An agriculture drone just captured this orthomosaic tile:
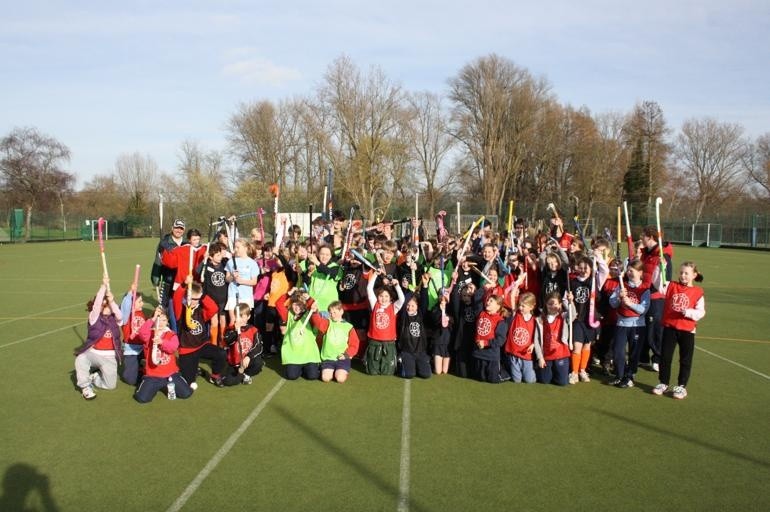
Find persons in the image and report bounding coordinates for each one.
[73,272,124,401]
[133,305,194,404]
[650,256,707,401]
[151,207,673,391]
[119,285,149,387]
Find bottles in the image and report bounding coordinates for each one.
[167,376,178,401]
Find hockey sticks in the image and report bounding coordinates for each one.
[151,282,166,366]
[565,266,575,350]
[570,196,579,237]
[445,198,514,292]
[309,203,312,272]
[200,215,212,284]
[218,215,240,287]
[280,216,286,241]
[588,257,600,329]
[98,217,110,293]
[604,227,627,310]
[340,191,422,286]
[517,218,529,291]
[208,210,267,227]
[235,293,244,374]
[573,214,590,252]
[185,245,197,330]
[129,265,140,339]
[655,197,666,289]
[546,202,563,234]
[440,253,449,328]
[300,274,330,336]
[257,207,266,268]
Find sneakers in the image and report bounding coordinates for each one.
[650,383,669,396]
[209,376,225,388]
[672,385,688,400]
[568,372,579,385]
[81,384,97,401]
[650,363,659,372]
[242,374,253,385]
[89,369,101,383]
[594,367,610,380]
[614,376,635,389]
[579,371,591,383]
[607,375,626,386]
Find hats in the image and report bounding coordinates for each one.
[172,217,185,229]
